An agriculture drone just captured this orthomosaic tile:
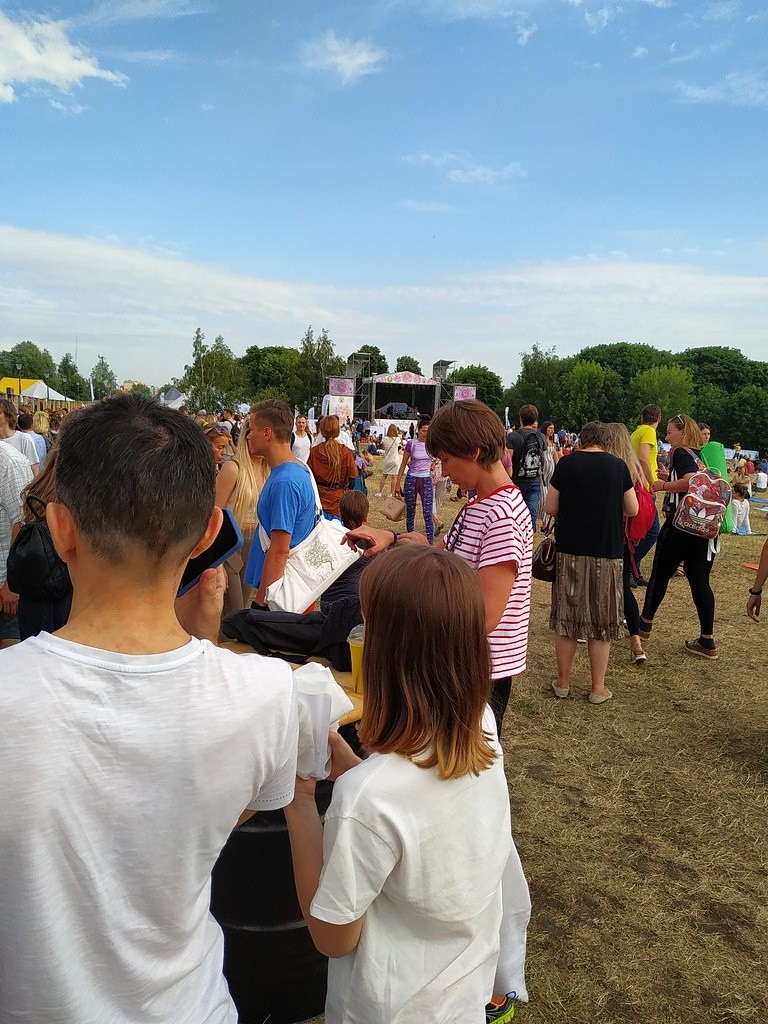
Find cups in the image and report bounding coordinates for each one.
[346,624,364,694]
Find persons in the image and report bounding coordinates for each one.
[284,545,510,1024]
[1,394,301,1024]
[545,421,640,705]
[1,398,768,664]
[341,399,532,1024]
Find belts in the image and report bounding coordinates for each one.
[316,481,341,489]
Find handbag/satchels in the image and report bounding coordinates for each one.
[263,515,366,615]
[379,491,406,522]
[532,532,556,582]
[220,608,326,664]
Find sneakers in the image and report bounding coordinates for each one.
[485,995,515,1024]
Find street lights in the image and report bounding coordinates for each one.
[44,369,51,406]
[86,383,108,403]
[61,374,67,405]
[15,358,24,405]
[76,380,80,407]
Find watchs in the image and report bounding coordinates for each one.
[749,587,762,595]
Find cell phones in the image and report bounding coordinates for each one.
[347,532,376,550]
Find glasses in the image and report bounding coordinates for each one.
[676,412,685,425]
[208,426,229,433]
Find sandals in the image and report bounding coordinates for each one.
[629,643,647,665]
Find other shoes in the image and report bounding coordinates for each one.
[588,685,612,704]
[685,638,718,659]
[434,521,444,537]
[374,490,381,497]
[552,678,570,697]
[576,635,587,643]
[623,619,650,641]
[446,490,450,496]
[630,572,649,588]
[390,493,392,496]
[674,563,686,577]
[450,497,459,502]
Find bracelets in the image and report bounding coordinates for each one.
[663,481,666,491]
[389,530,398,547]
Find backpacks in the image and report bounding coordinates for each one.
[668,445,733,539]
[7,496,71,597]
[622,463,658,579]
[515,429,544,480]
[226,419,241,447]
